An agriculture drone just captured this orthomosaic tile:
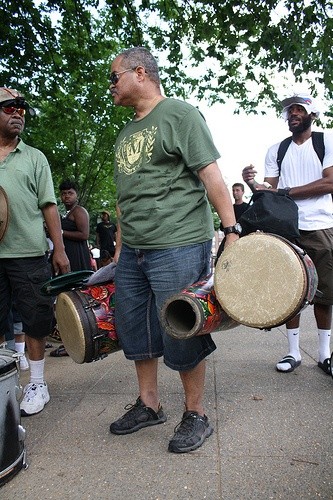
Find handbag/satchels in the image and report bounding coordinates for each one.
[236,189,301,239]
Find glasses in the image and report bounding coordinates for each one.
[109,68,147,85]
[2,106,26,116]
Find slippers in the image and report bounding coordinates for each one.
[276,356,301,373]
[317,357,332,375]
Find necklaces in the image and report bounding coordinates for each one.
[62,203,77,219]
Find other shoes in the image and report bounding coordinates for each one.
[50,346,69,356]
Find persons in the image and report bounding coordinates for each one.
[107,47,240,453]
[242,94,333,376]
[0,87,71,416]
[12,179,117,371]
[220,183,250,231]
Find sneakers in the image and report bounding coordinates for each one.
[168,411,213,452]
[109,396,167,434]
[19,382,51,416]
[20,358,29,370]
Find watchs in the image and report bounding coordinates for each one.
[224,223,242,235]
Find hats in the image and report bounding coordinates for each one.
[0,87,29,107]
[281,91,320,114]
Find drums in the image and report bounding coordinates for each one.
[0,342,29,488]
[54,280,123,364]
[0,185,10,242]
[160,273,240,340]
[215,231,318,330]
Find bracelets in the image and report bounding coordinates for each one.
[284,187,290,195]
